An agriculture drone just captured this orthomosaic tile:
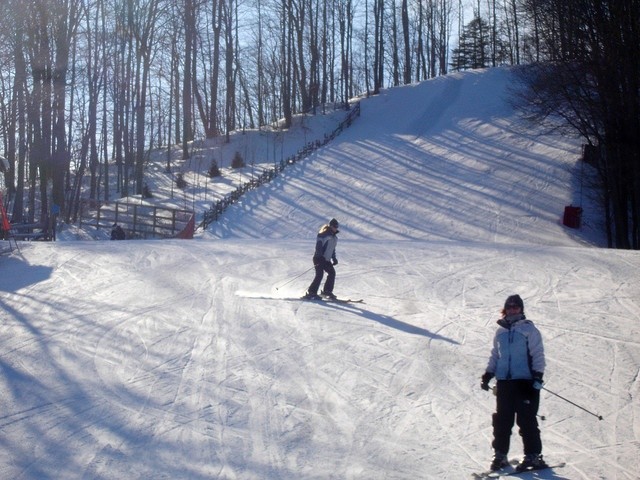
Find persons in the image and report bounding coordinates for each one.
[110,223,126,240]
[305,218,340,302]
[481,294,546,472]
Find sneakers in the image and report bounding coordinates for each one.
[323,291,337,299]
[309,293,322,300]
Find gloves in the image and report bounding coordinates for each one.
[481,373,492,391]
[534,373,543,390]
[331,258,338,267]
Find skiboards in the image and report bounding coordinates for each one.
[283,297,364,303]
[471,459,566,478]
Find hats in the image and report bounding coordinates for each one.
[506,294,522,305]
[329,218,338,226]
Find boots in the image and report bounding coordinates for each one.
[490,449,509,470]
[518,451,545,470]
